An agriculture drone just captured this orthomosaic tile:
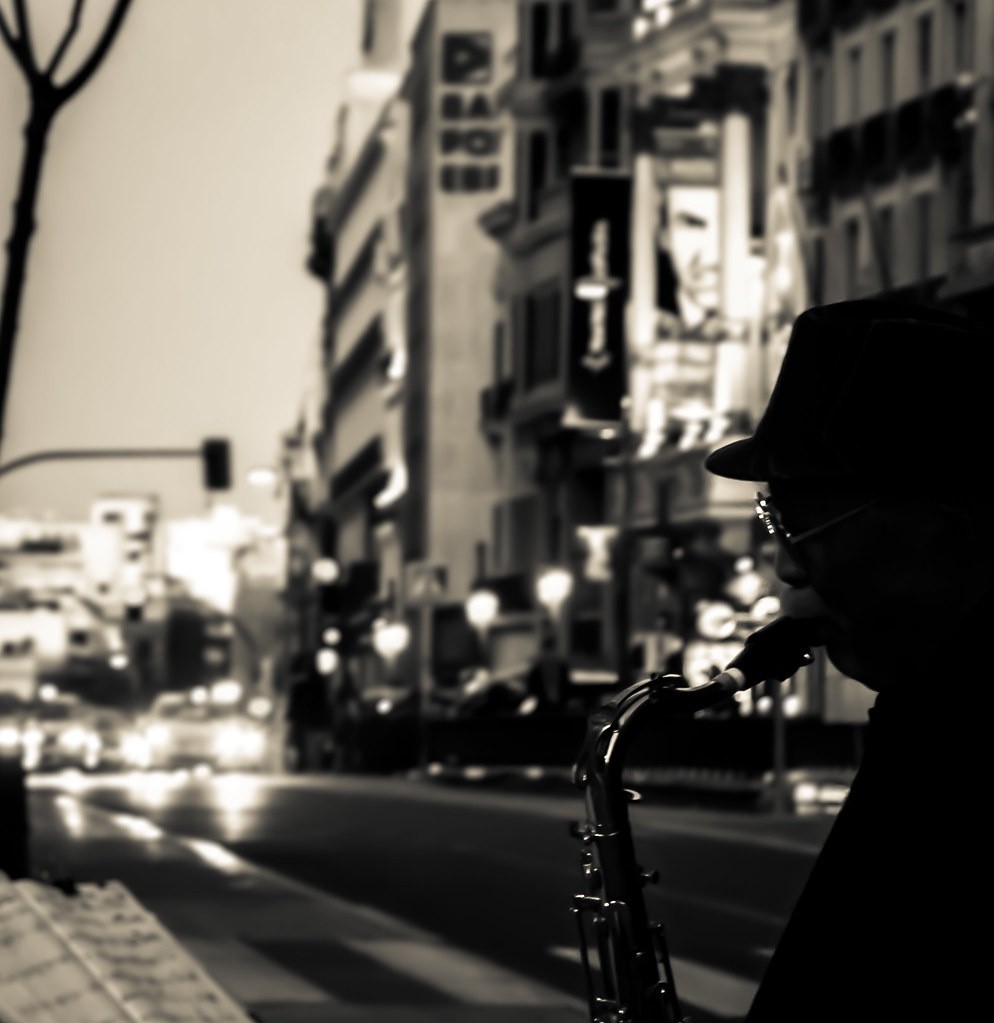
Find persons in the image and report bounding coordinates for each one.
[706,281,994,1023]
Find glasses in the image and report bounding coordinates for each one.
[754,488,866,545]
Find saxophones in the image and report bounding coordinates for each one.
[563,609,828,1023]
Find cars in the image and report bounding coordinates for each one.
[0,689,265,774]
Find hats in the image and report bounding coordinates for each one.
[705,297,994,481]
